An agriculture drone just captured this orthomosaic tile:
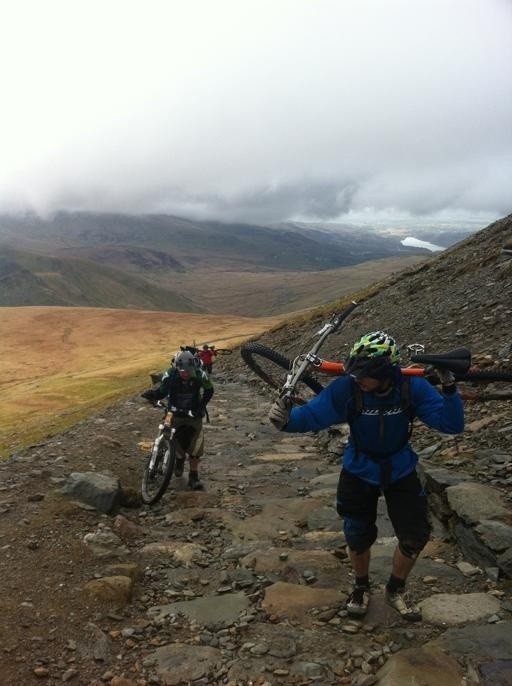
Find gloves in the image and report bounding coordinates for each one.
[433,349,455,387]
[269,397,294,431]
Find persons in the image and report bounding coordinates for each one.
[266,330,465,621]
[141,350,215,491]
[196,344,217,375]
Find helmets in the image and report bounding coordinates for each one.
[343,330,402,379]
[175,350,199,372]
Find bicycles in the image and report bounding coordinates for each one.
[136,392,202,509]
[181,340,232,358]
[234,294,511,439]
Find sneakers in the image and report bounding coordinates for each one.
[382,584,422,621]
[189,479,203,490]
[346,587,371,615]
[175,452,185,477]
